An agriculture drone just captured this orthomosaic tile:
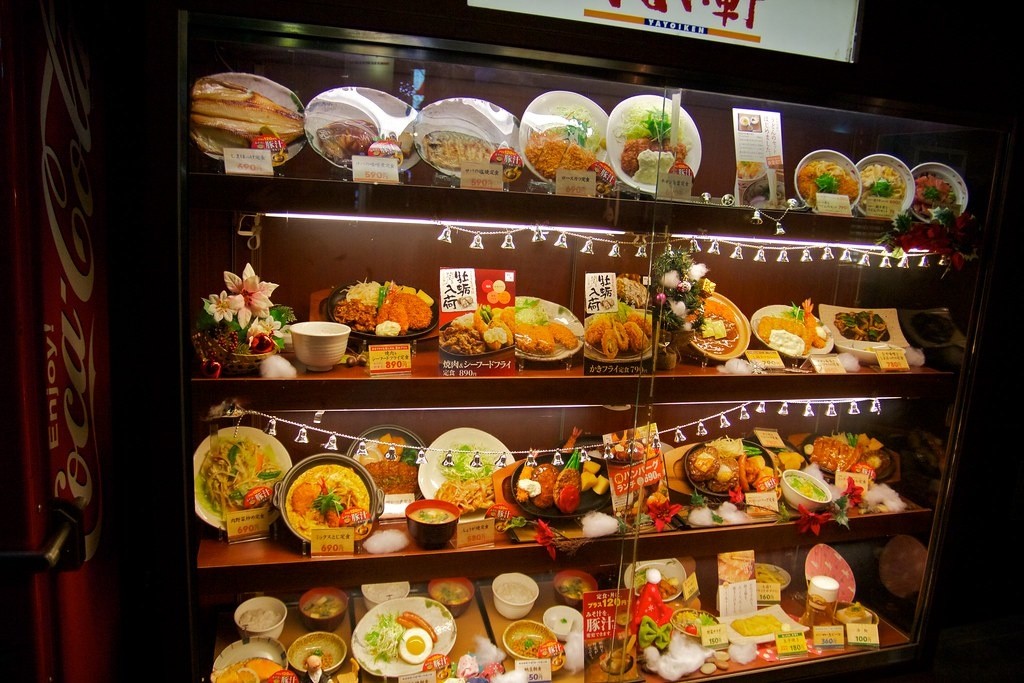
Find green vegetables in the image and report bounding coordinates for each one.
[441,444,494,480]
[791,479,828,502]
[696,613,715,634]
[312,488,345,518]
[228,441,281,499]
[364,611,405,662]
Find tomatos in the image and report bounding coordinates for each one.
[686,624,697,635]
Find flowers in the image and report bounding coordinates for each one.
[201,262,297,355]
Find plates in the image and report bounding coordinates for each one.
[191,73,701,193]
[319,281,440,340]
[624,543,879,648]
[439,319,516,362]
[212,638,288,683]
[674,432,896,497]
[351,596,457,677]
[583,308,652,363]
[686,292,964,365]
[193,425,610,541]
[605,438,662,464]
[514,296,586,361]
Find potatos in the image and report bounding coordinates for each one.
[580,461,610,495]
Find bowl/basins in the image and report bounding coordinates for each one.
[405,498,461,550]
[493,568,598,661]
[234,596,287,639]
[781,469,832,512]
[737,164,785,210]
[288,632,347,677]
[299,588,349,631]
[428,575,475,619]
[793,149,968,223]
[290,321,352,372]
[361,582,410,610]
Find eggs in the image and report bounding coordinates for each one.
[398,627,433,664]
[646,568,661,584]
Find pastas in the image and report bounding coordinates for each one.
[201,438,279,514]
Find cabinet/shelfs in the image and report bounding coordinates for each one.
[91,0,1004,683]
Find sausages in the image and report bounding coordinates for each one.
[398,611,438,642]
